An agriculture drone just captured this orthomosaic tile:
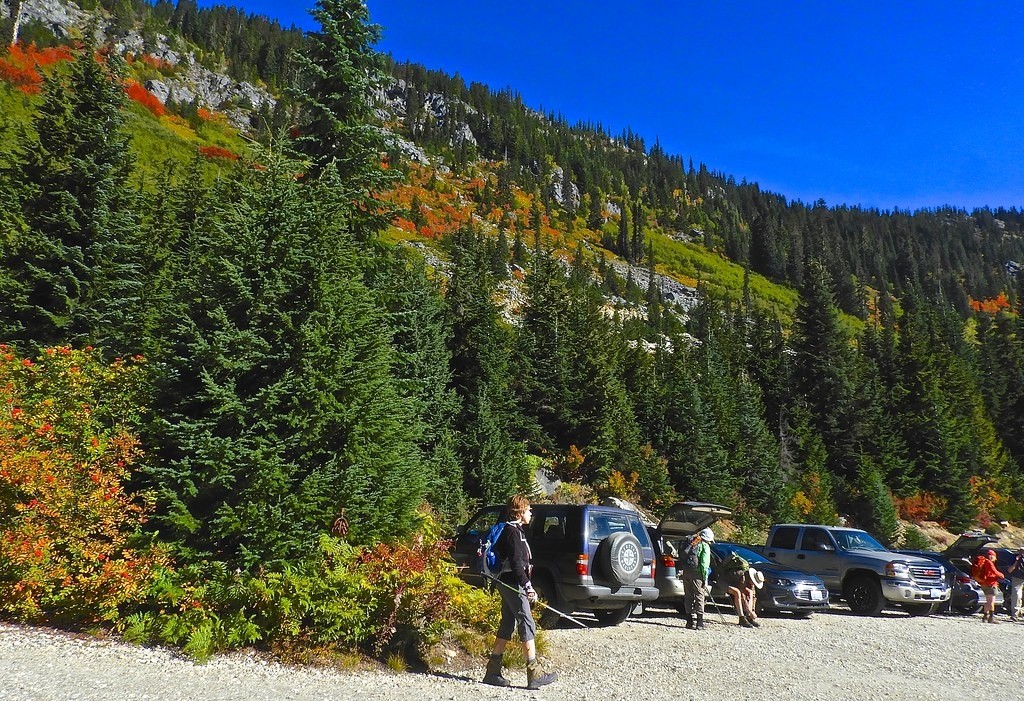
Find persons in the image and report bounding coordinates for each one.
[979,550,1006,623]
[482,495,558,690]
[710,555,765,629]
[678,527,716,630]
[1007,546,1024,622]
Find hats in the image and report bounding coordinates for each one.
[749,567,764,589]
[699,526,715,544]
[986,550,996,557]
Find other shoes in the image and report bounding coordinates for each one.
[1009,616,1019,621]
[982,614,987,622]
[988,616,999,624]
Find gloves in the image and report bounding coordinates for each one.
[522,581,538,603]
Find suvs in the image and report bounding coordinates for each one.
[444,501,660,630]
[763,523,952,617]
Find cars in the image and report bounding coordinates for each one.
[614,501,734,614]
[707,542,829,618]
[974,546,1024,614]
[887,527,1005,616]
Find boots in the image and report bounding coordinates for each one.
[525,658,558,689]
[685,614,693,629]
[746,616,760,627]
[696,614,704,630]
[739,616,753,628]
[483,654,510,687]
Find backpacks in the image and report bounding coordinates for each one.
[470,521,522,575]
[972,555,991,585]
[678,536,701,569]
[716,551,750,576]
